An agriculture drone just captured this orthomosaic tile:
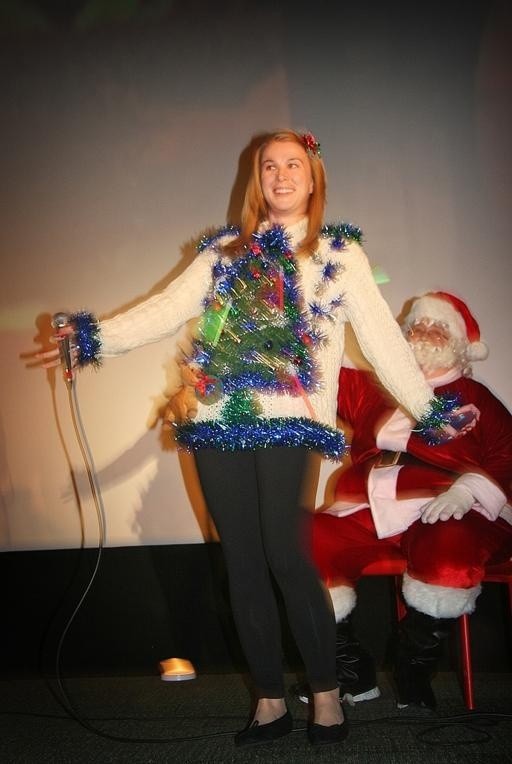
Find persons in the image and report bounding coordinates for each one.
[27,126,482,752]
[289,289,509,712]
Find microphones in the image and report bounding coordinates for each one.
[50,312,72,383]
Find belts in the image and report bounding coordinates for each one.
[360,448,429,470]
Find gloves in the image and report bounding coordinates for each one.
[419,486,471,525]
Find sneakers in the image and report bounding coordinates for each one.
[392,657,438,713]
[292,667,382,705]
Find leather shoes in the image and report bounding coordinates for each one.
[306,699,349,748]
[233,702,294,746]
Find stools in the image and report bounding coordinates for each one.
[358,547,512,714]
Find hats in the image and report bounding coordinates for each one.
[405,290,489,364]
